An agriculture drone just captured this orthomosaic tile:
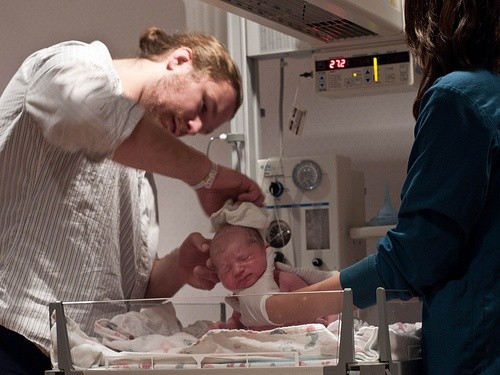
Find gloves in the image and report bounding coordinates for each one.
[275,262,338,286]
[224,247,285,327]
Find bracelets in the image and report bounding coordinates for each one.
[189,161,219,191]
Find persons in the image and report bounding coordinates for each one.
[224,0,500,375]
[207,224,330,332]
[0,25,264,375]
